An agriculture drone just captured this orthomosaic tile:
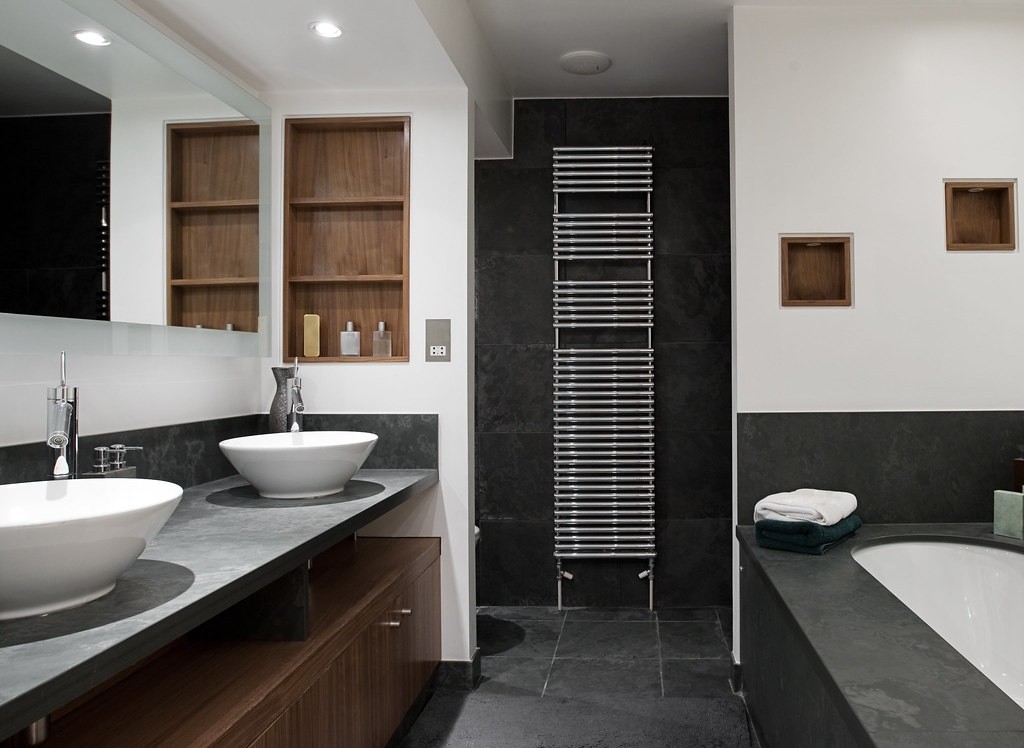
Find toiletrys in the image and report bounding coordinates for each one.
[339,321,362,357]
[108,444,144,479]
[371,321,392,358]
[82,446,122,478]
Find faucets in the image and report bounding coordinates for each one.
[286,357,306,433]
[43,349,81,480]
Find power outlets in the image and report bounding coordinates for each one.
[426,319,452,362]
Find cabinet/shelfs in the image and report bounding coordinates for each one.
[283,117,409,363]
[162,117,261,333]
[35,538,443,748]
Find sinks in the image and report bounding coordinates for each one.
[218,429,379,500]
[1,476,184,624]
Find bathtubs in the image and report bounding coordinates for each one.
[733,505,1024,748]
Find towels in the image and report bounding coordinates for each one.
[752,516,864,555]
[752,487,858,526]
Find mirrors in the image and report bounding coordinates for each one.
[1,0,261,333]
